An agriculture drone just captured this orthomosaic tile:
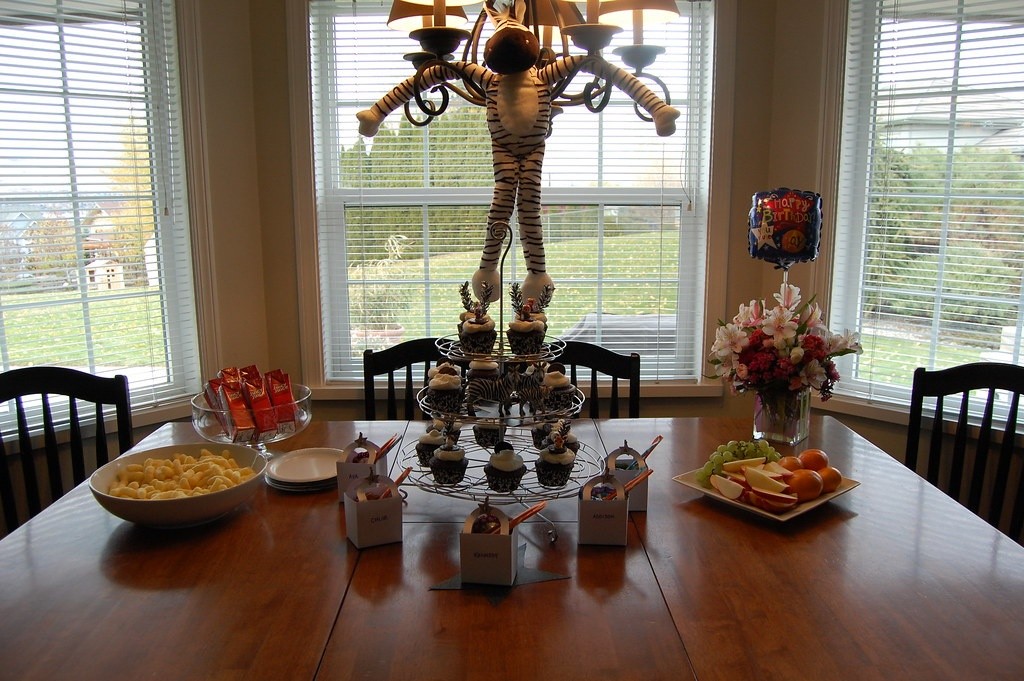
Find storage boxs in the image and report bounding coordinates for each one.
[335,430,650,588]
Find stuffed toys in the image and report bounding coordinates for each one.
[356,18,682,311]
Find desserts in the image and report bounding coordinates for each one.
[427,357,576,414]
[415,416,580,493]
[456,280,553,355]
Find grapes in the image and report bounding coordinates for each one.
[695,435,781,489]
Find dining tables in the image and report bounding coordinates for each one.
[0,421,1021,681]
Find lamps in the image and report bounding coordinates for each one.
[386,0,681,138]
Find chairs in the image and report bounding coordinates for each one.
[904,360,1024,546]
[544,340,640,417]
[364,337,473,423]
[0,364,134,536]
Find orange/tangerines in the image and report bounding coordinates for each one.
[779,448,842,502]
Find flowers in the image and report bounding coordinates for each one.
[702,281,863,432]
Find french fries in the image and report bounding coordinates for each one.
[107,448,256,500]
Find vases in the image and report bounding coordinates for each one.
[752,384,811,445]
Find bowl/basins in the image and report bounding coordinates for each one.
[191,382,313,458]
[87,442,267,530]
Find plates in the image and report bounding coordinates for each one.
[264,447,344,492]
[670,456,861,524]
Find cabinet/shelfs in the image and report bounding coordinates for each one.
[395,220,608,545]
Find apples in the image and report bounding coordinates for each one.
[710,456,798,513]
